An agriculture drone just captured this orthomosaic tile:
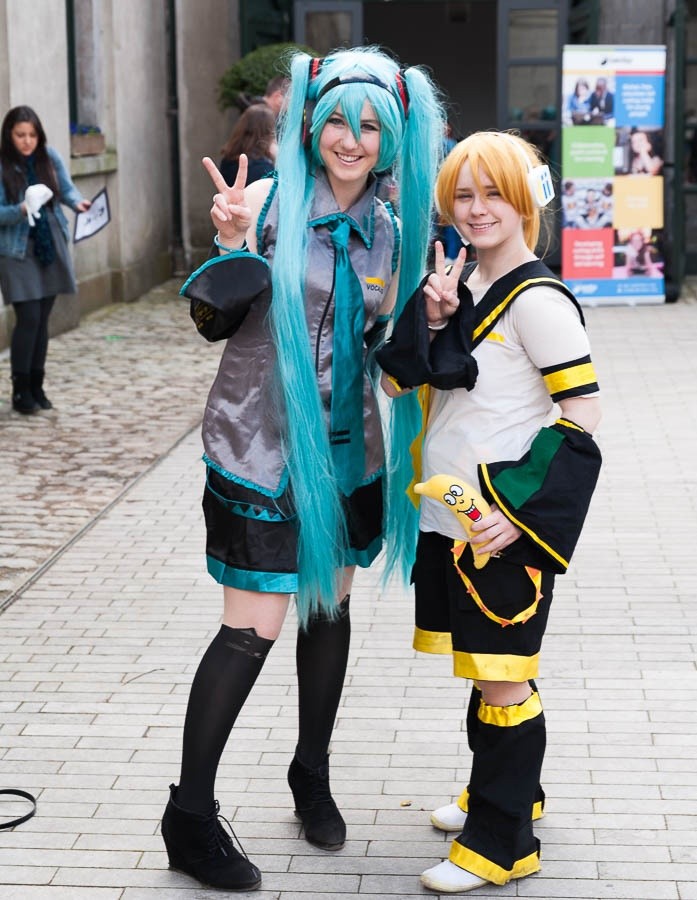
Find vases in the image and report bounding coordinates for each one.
[71,135,106,158]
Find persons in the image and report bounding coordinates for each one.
[629,133,664,175]
[562,180,612,227]
[0,106,93,413]
[220,102,274,189]
[159,49,443,888]
[388,132,600,896]
[252,75,288,116]
[570,79,613,126]
[623,232,663,277]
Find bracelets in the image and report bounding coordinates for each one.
[428,320,449,331]
[214,235,247,254]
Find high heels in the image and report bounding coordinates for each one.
[288,743,346,850]
[162,781,261,891]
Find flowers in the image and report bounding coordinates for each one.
[70,124,101,135]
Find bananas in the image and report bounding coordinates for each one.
[413,473,492,569]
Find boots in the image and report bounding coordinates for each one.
[11,375,40,416]
[34,371,51,409]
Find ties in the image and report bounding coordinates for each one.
[328,217,368,498]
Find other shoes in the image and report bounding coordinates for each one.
[430,801,470,831]
[421,860,489,892]
